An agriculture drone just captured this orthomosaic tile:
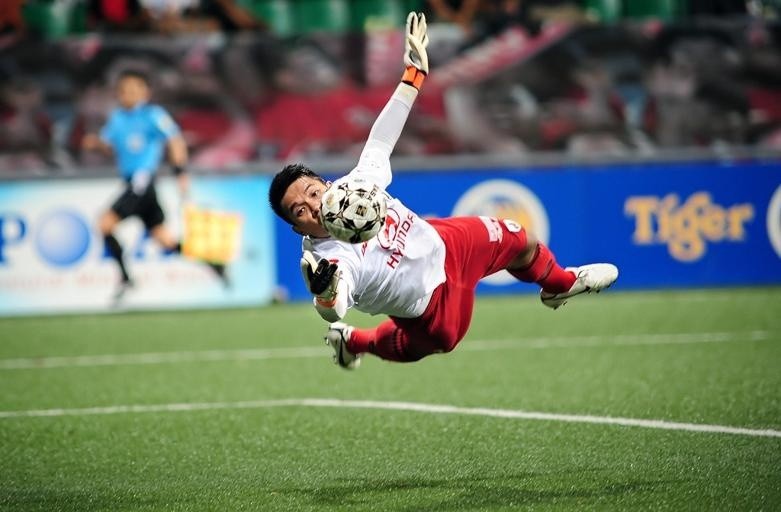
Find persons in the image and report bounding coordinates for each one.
[83,72,234,306]
[268,11,620,369]
[1,0,780,177]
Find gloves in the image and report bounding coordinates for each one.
[403,12,429,76]
[300,250,342,301]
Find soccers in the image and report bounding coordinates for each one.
[320,178,388,243]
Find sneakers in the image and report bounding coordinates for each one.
[540,263,619,309]
[324,322,361,370]
